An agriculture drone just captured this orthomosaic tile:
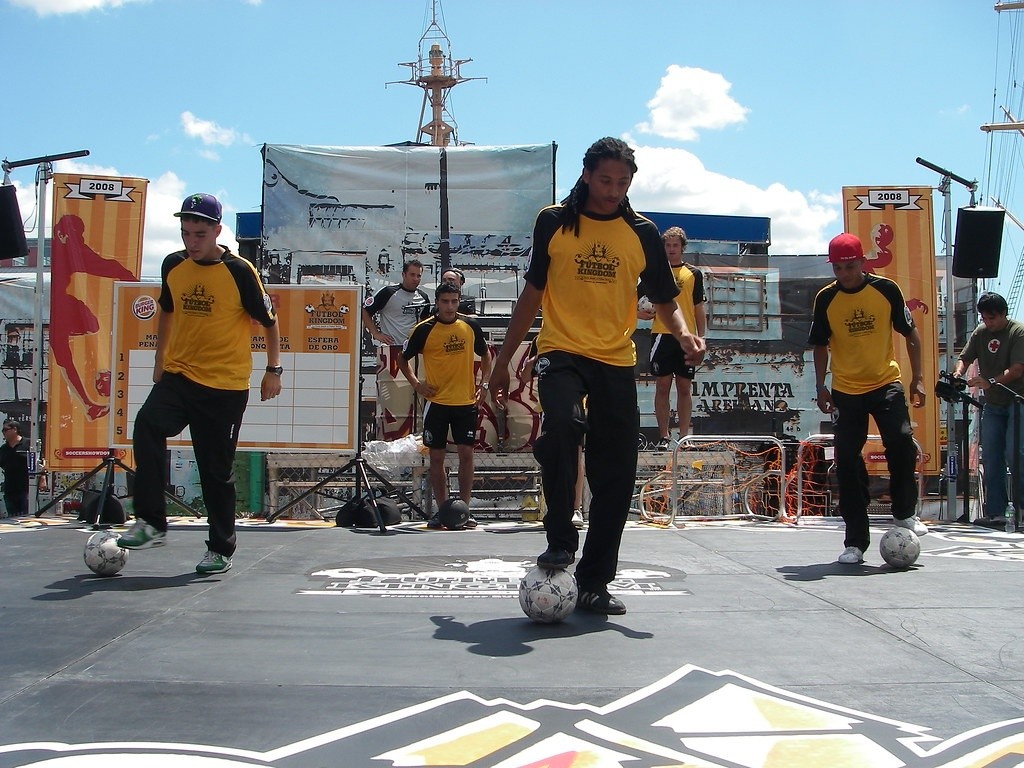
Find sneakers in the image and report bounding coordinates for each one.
[577,581,627,614]
[537,547,576,569]
[895,514,928,537]
[839,547,863,562]
[196,550,233,573]
[117,518,167,550]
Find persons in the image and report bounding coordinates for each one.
[636,227,706,451]
[430,269,474,316]
[363,260,430,345]
[116,193,283,574]
[490,136,706,615]
[953,291,1024,531]
[396,283,492,528]
[0,421,31,517]
[520,334,587,526]
[807,233,928,564]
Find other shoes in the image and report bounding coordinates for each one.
[427,512,444,527]
[465,513,477,527]
[571,506,583,525]
[974,515,1007,527]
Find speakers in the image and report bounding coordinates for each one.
[952,205,1005,278]
[0,185,30,260]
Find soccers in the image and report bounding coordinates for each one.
[879,525,922,568]
[439,498,470,529]
[519,564,579,625]
[84,531,129,576]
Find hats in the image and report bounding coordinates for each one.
[442,268,465,285]
[826,232,865,263]
[174,192,222,221]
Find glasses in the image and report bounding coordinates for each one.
[2,428,13,432]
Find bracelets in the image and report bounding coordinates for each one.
[816,385,827,392]
[701,336,705,339]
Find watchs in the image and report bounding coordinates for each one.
[987,377,996,388]
[479,382,489,389]
[266,366,283,376]
